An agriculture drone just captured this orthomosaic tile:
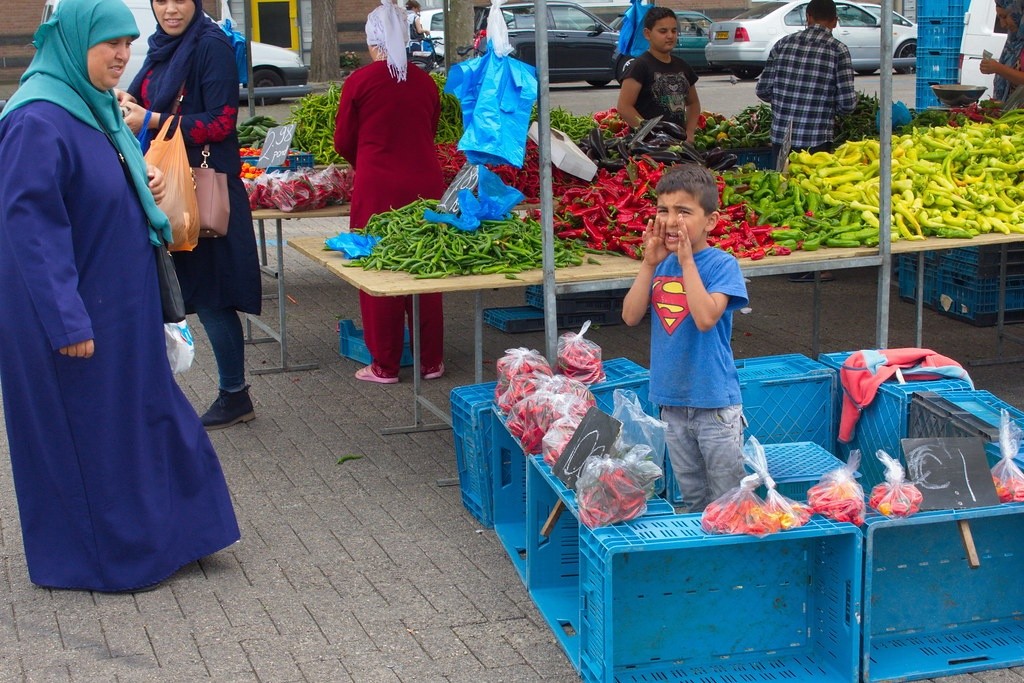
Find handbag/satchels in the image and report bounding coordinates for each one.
[990,407,1024,503]
[700,473,781,539]
[557,319,606,385]
[143,115,200,252]
[409,15,424,39]
[241,162,354,213]
[868,449,923,520]
[806,449,866,527]
[495,346,669,523]
[741,435,815,531]
[192,144,231,237]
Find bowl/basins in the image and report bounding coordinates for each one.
[929,81,988,105]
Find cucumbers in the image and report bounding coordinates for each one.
[236,114,283,149]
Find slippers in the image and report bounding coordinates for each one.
[355,365,399,383]
[423,361,445,380]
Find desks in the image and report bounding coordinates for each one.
[281,188,1024,490]
[252,189,350,277]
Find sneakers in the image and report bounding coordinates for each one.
[199,384,255,430]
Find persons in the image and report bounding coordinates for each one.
[617,7,701,144]
[113,0,263,431]
[0,0,243,592]
[980,0,1024,102]
[334,0,447,384]
[403,0,431,52]
[756,0,857,281]
[622,164,751,514]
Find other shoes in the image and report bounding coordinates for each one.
[787,271,834,283]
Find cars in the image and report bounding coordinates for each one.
[608,11,712,70]
[475,1,635,87]
[419,6,515,65]
[858,4,916,27]
[705,0,917,80]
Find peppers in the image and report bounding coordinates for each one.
[281,72,467,189]
[869,482,922,516]
[336,453,363,464]
[496,332,605,465]
[527,101,630,146]
[891,93,1024,242]
[237,146,356,213]
[578,443,664,529]
[324,154,620,281]
[695,87,881,154]
[520,133,881,260]
[701,484,864,536]
[991,474,1024,502]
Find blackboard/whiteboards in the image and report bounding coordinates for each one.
[256,124,296,168]
[901,438,1000,511]
[551,406,624,491]
[628,115,664,147]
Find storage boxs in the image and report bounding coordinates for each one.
[915,0,971,116]
[714,147,775,174]
[239,150,313,174]
[338,242,1024,683]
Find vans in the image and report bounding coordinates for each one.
[42,1,309,107]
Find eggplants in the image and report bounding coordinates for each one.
[578,115,737,174]
